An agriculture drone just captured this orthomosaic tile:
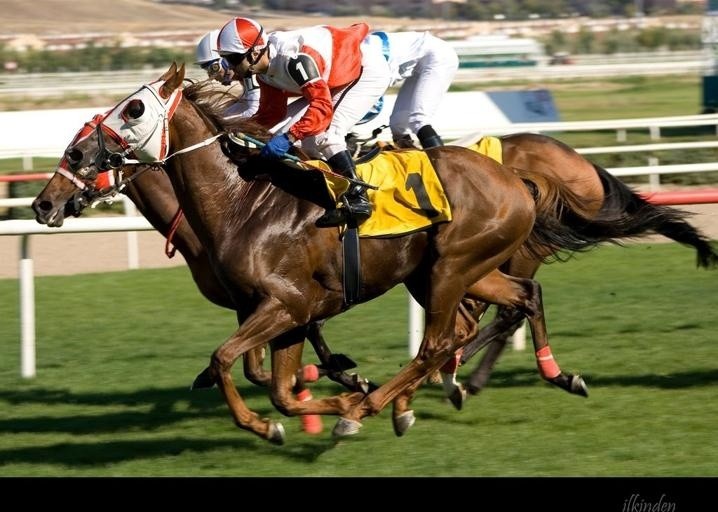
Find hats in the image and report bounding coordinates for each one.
[214,17,268,57]
[193,30,222,65]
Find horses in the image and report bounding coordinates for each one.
[28,105,718,399]
[63,58,700,446]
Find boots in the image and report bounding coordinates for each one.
[315,149,373,229]
[421,135,443,150]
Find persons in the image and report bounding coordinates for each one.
[212,16,392,229]
[191,26,460,149]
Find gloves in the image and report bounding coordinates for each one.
[262,132,292,160]
